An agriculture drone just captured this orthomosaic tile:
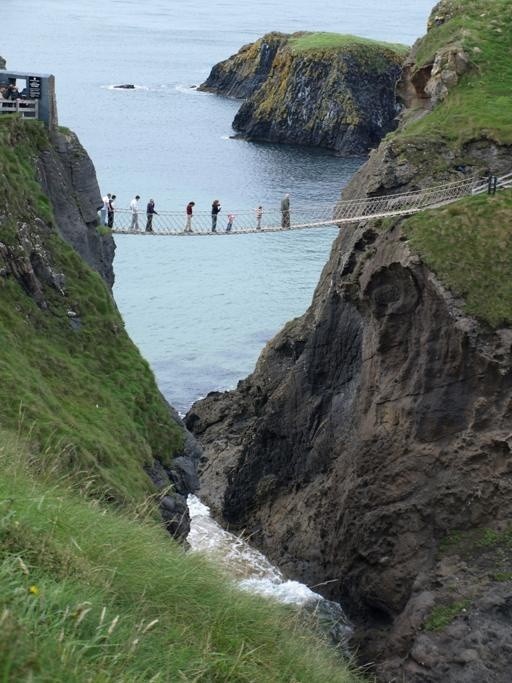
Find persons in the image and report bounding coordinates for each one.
[128,194,143,230]
[101,193,111,223]
[210,199,222,231]
[145,198,160,231]
[225,212,236,232]
[106,194,118,227]
[281,192,292,228]
[0,83,31,114]
[255,205,264,228]
[182,200,195,232]
